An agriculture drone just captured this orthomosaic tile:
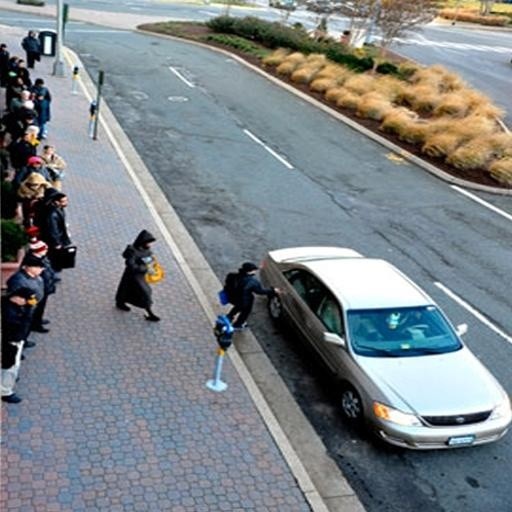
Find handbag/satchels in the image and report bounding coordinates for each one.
[144,255,163,282]
[48,245,78,269]
[219,290,228,304]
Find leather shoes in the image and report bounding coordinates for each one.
[1,275,61,405]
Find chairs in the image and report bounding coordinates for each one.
[323,303,359,336]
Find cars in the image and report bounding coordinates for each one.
[261,244,512,451]
[268,0,368,17]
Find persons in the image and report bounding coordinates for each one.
[113,228,160,322]
[218,262,275,330]
[0,43,77,402]
[21,30,41,68]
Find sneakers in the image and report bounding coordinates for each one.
[144,314,159,321]
[116,302,130,311]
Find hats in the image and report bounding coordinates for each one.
[29,236,48,254]
